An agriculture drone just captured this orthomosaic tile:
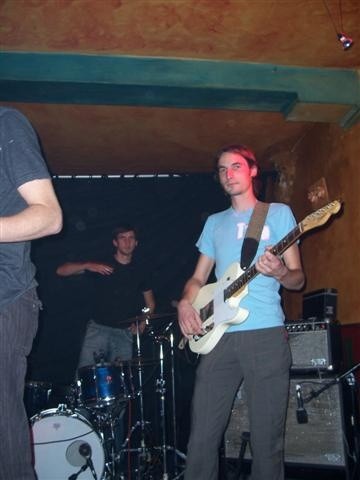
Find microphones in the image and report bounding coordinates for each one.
[79,444,90,457]
[238,431,250,471]
[295,383,308,425]
[161,319,175,334]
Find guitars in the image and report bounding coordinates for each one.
[188,200,341,355]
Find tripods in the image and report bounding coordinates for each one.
[102,317,188,480]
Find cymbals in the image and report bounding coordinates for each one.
[121,313,175,323]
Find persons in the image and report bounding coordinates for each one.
[55,226,156,382]
[176,144,305,480]
[0,104,63,480]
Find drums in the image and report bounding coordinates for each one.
[28,407,106,480]
[78,361,140,408]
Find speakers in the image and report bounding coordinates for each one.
[224,371,357,471]
